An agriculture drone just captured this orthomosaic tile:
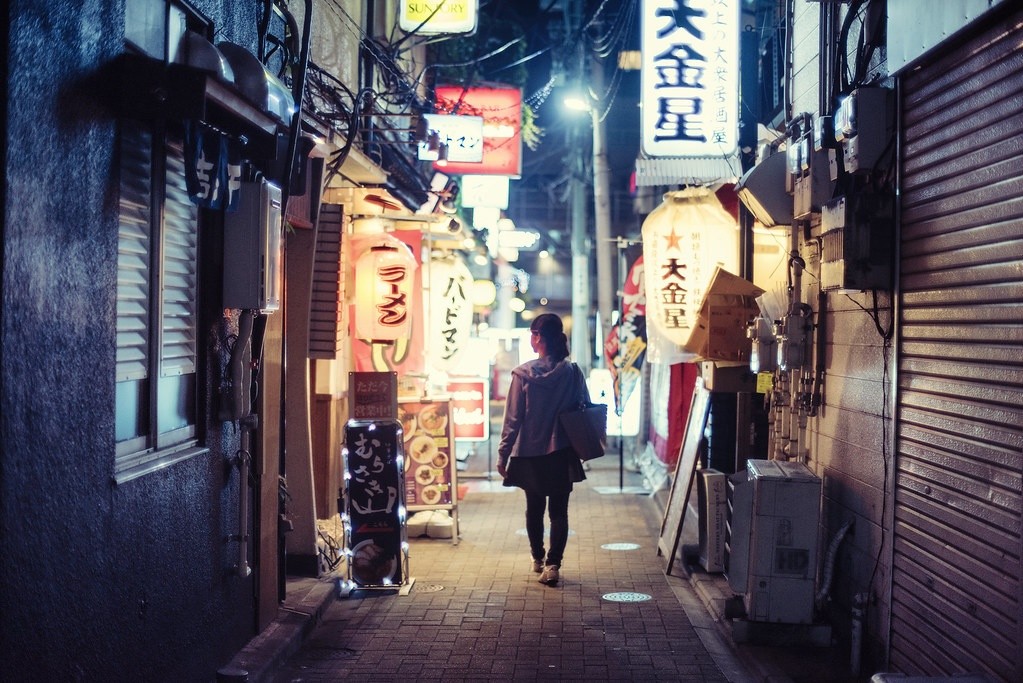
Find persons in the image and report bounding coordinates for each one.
[496,313,591,583]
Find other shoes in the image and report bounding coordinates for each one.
[531,559,545,572]
[535,565,560,584]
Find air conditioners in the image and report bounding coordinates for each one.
[732,452,827,626]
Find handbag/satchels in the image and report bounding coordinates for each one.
[559,362,608,461]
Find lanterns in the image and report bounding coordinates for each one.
[354,246,411,345]
[643,186,739,348]
[421,255,475,369]
